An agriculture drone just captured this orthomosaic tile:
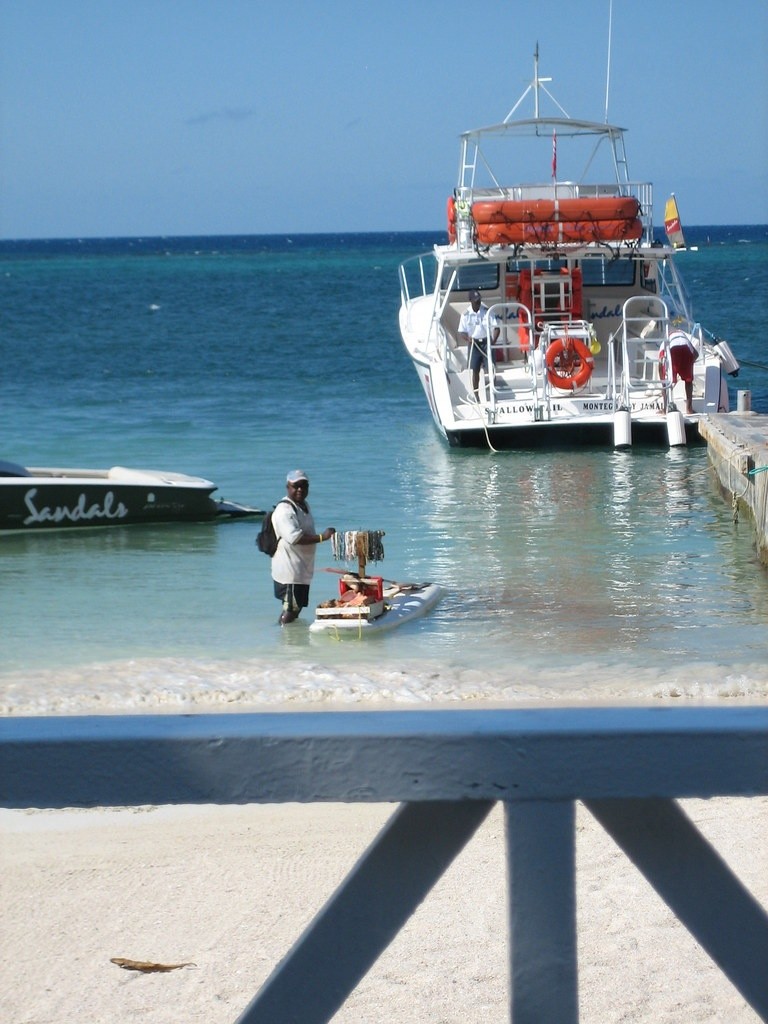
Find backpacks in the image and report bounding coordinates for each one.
[256,500,298,558]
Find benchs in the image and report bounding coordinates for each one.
[610,310,669,382]
[444,323,470,372]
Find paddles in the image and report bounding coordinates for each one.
[320,565,405,587]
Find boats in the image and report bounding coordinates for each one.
[0,458,269,531]
[396,0,732,450]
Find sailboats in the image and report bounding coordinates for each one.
[663,193,688,252]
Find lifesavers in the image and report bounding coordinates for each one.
[446,198,460,246]
[544,337,595,390]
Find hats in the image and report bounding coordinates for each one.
[286,470,309,483]
[468,290,482,302]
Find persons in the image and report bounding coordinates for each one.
[457,291,500,405]
[656,331,700,415]
[271,470,336,625]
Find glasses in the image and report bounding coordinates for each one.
[289,483,309,489]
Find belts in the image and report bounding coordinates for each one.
[475,338,485,343]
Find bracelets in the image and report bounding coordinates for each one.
[320,534,322,542]
